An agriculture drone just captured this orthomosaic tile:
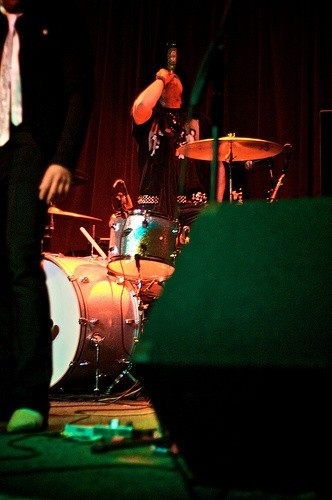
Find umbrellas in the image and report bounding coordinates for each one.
[128,197,332,500]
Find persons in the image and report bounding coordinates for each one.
[130,67,204,227]
[246,128,295,203]
[0,0,95,434]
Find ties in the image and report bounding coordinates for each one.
[0,6,23,146]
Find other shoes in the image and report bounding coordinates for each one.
[8,408,44,433]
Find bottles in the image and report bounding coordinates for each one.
[166,42,177,76]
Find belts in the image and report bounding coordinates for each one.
[137,191,207,206]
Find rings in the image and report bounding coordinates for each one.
[60,176,67,183]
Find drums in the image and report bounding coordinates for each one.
[41,251,141,394]
[104,210,177,279]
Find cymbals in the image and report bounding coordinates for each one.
[48,203,101,220]
[176,137,281,162]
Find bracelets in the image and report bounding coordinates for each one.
[156,76,165,88]
